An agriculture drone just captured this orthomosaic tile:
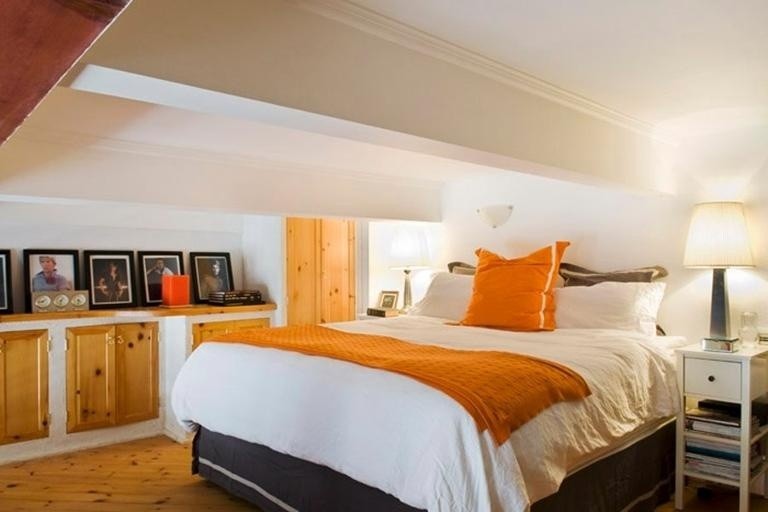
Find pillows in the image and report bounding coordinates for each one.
[457,240,572,333]
[406,271,475,323]
[554,282,667,337]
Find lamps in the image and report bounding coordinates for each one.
[475,205,514,229]
[389,226,435,315]
[682,201,758,352]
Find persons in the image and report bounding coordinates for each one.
[148,258,174,276]
[31,255,71,291]
[99,261,123,302]
[202,259,223,295]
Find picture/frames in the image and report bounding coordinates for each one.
[0,249,15,315]
[83,250,137,310]
[379,291,399,311]
[136,251,186,308]
[22,249,81,314]
[189,252,235,305]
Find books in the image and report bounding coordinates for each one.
[209,289,261,305]
[368,306,399,317]
[683,398,764,485]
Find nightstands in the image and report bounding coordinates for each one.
[675,338,768,512]
[359,311,405,321]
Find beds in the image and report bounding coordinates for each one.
[169,261,688,512]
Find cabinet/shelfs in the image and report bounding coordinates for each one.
[191,317,271,351]
[0,328,49,445]
[66,322,160,434]
[286,215,356,325]
